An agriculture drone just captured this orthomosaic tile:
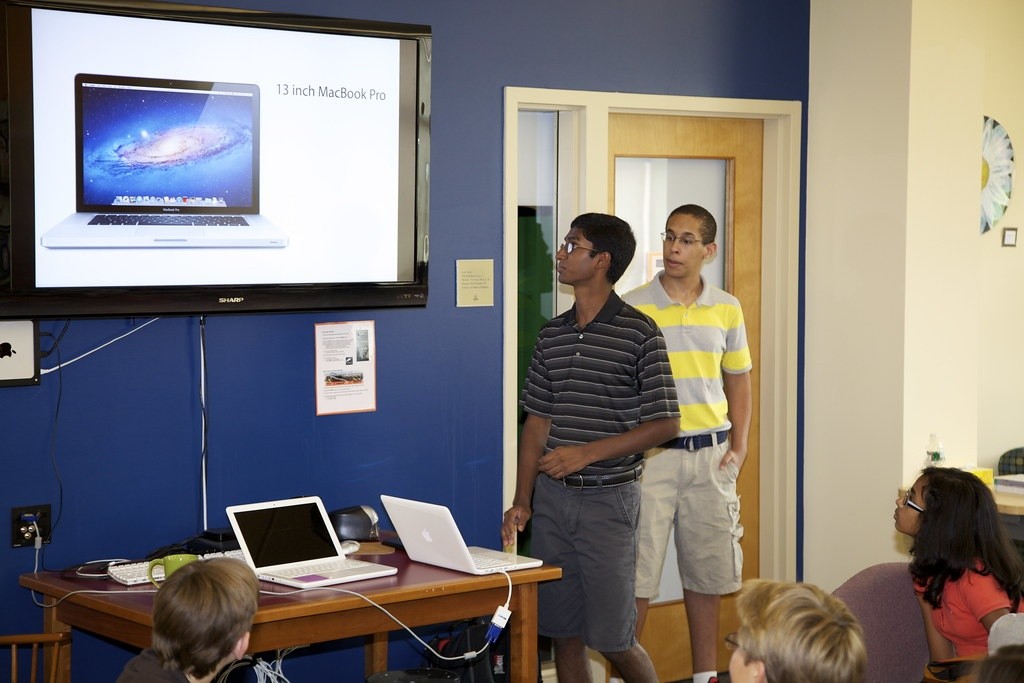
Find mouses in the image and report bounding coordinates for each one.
[340,540,358,555]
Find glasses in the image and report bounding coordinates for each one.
[903,488,925,513]
[559,243,601,254]
[660,232,708,245]
[724,631,748,653]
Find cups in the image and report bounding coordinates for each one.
[147,553,198,590]
[971,469,994,490]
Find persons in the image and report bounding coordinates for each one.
[979,644,1024,683]
[728,578,867,683]
[622,204,752,683]
[895,466,1024,683]
[116,558,259,683]
[503,213,681,683]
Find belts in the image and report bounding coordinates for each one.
[560,464,643,490]
[657,430,727,452]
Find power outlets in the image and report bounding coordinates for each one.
[10,504,53,548]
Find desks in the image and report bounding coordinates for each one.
[19,530,563,683]
[988,486,1024,515]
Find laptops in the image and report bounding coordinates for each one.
[41,72,289,250]
[225,496,399,589]
[381,495,544,575]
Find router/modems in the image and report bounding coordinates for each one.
[0,319,42,387]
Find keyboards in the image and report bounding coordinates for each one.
[108,550,246,585]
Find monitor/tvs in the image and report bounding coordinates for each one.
[0,0,432,319]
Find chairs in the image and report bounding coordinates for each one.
[1,630,71,682]
